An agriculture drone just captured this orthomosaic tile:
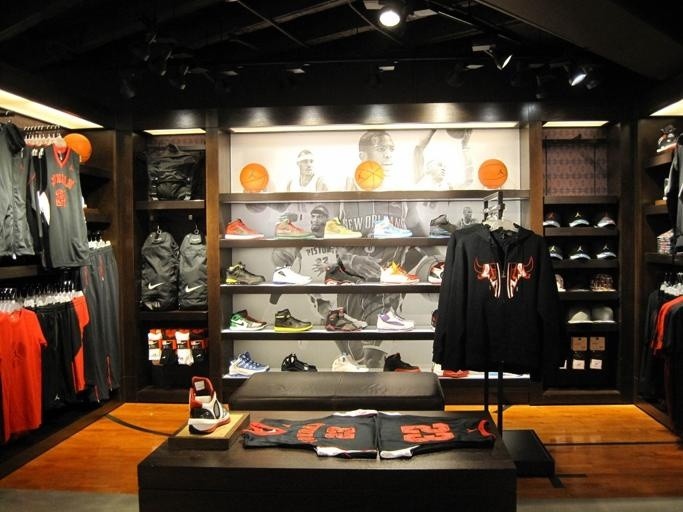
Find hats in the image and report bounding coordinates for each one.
[593,210,616,229]
[567,308,593,324]
[595,242,618,260]
[567,209,589,229]
[568,241,592,261]
[592,306,616,324]
[554,273,566,292]
[542,211,561,228]
[590,273,616,291]
[548,245,564,261]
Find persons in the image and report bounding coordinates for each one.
[273,204,366,371]
[454,205,480,229]
[316,131,446,372]
[249,149,341,224]
[415,128,479,191]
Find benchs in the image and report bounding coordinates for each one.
[229,372,445,411]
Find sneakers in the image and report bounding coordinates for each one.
[326,307,367,331]
[383,352,420,372]
[228,309,267,332]
[229,351,270,377]
[275,219,315,239]
[226,262,264,285]
[380,262,421,283]
[282,353,318,373]
[377,311,414,330]
[188,376,231,434]
[373,215,413,238]
[332,356,364,372]
[272,265,312,285]
[429,214,458,239]
[427,260,447,282]
[224,217,265,241]
[274,309,312,333]
[325,216,363,239]
[323,257,366,284]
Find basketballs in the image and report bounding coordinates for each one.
[239,162,270,193]
[354,160,385,190]
[62,133,92,164]
[478,159,509,190]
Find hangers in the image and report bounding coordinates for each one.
[0,281,84,314]
[86,229,111,252]
[21,124,82,162]
[654,270,683,288]
[2,111,14,124]
[481,191,519,235]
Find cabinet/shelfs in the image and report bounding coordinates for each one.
[73,164,114,228]
[544,137,619,388]
[220,126,522,378]
[642,148,681,256]
[136,410,516,512]
[135,144,207,390]
